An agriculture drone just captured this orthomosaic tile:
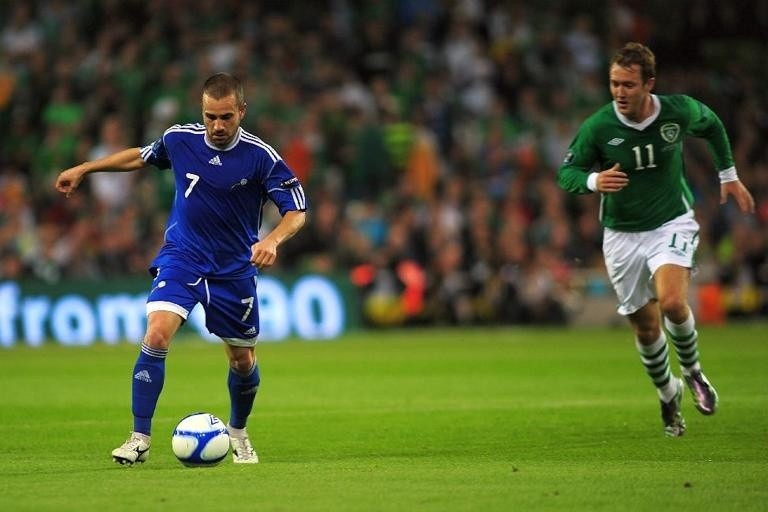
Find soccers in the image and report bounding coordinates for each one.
[171,413,230,467]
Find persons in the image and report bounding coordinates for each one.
[54,71,310,468]
[2,1,767,326]
[558,40,757,441]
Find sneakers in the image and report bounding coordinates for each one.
[110,430,152,467]
[683,368,719,416]
[655,374,687,440]
[225,419,260,465]
